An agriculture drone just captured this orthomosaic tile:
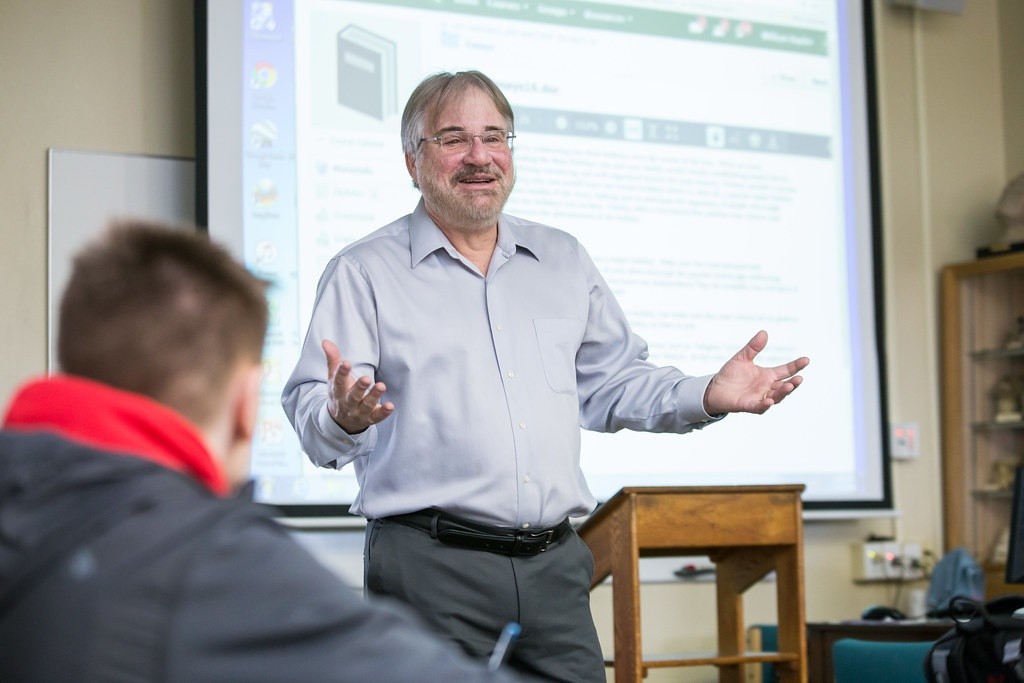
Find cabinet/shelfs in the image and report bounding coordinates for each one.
[940,255,1024,601]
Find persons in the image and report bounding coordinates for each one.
[282,71,810,683]
[0,221,524,683]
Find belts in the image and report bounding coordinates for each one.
[382,506,575,558]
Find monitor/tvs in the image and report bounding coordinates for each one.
[1005,463,1024,584]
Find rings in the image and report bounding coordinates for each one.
[762,393,767,399]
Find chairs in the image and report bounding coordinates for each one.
[745,624,778,683]
[832,638,935,683]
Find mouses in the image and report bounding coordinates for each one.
[862,604,906,621]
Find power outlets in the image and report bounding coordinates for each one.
[903,542,925,582]
[851,541,885,583]
[884,540,903,581]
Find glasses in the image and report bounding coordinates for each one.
[418,132,516,152]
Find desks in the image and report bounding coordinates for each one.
[576,483,808,683]
[807,620,954,683]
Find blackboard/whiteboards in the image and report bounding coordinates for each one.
[43,145,202,383]
